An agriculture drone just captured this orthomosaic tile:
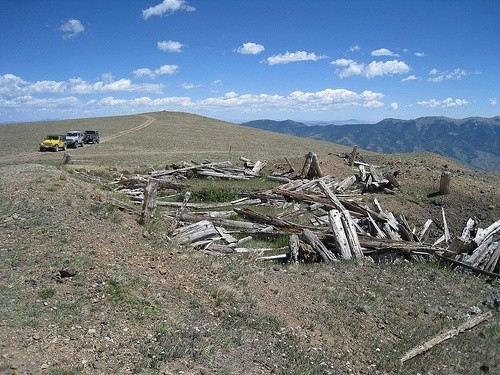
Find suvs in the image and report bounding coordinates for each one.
[66,131,85,149]
[82,129,100,144]
[38,134,68,152]
